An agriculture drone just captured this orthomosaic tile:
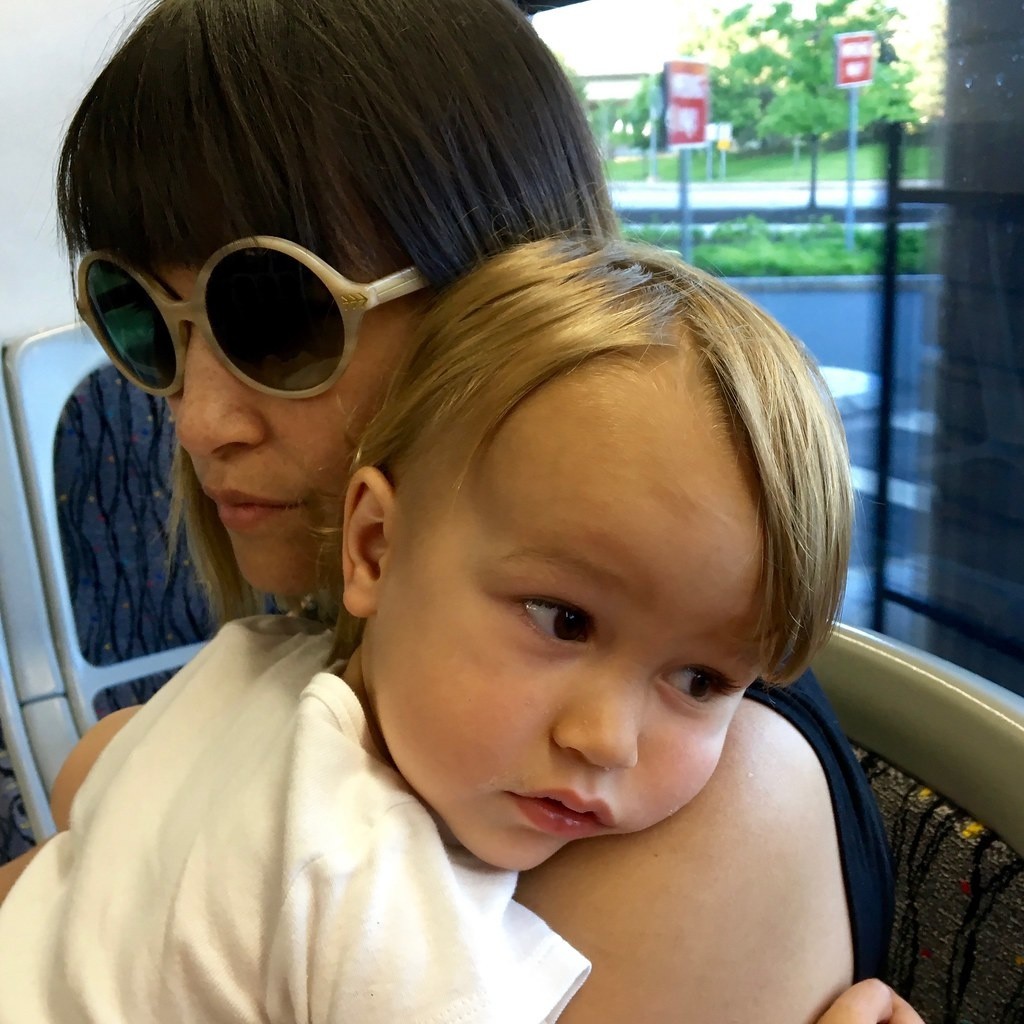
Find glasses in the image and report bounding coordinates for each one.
[73,236,426,399]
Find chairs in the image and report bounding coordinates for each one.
[812,619,1024,1024]
[1,321,280,853]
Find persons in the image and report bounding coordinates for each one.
[0,238,928,1023]
[0,0,892,1023]
